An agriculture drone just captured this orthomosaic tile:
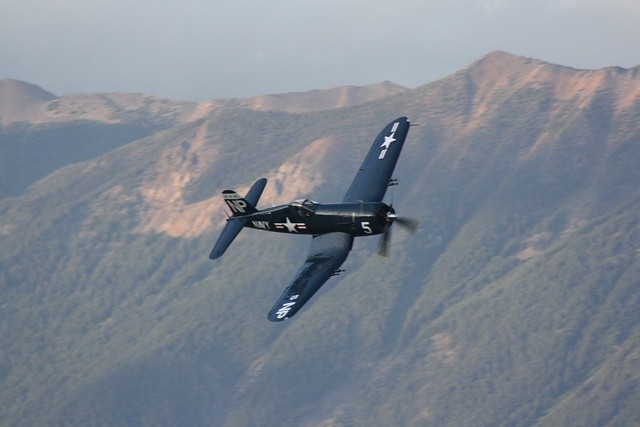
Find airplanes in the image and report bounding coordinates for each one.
[209,116,420,323]
